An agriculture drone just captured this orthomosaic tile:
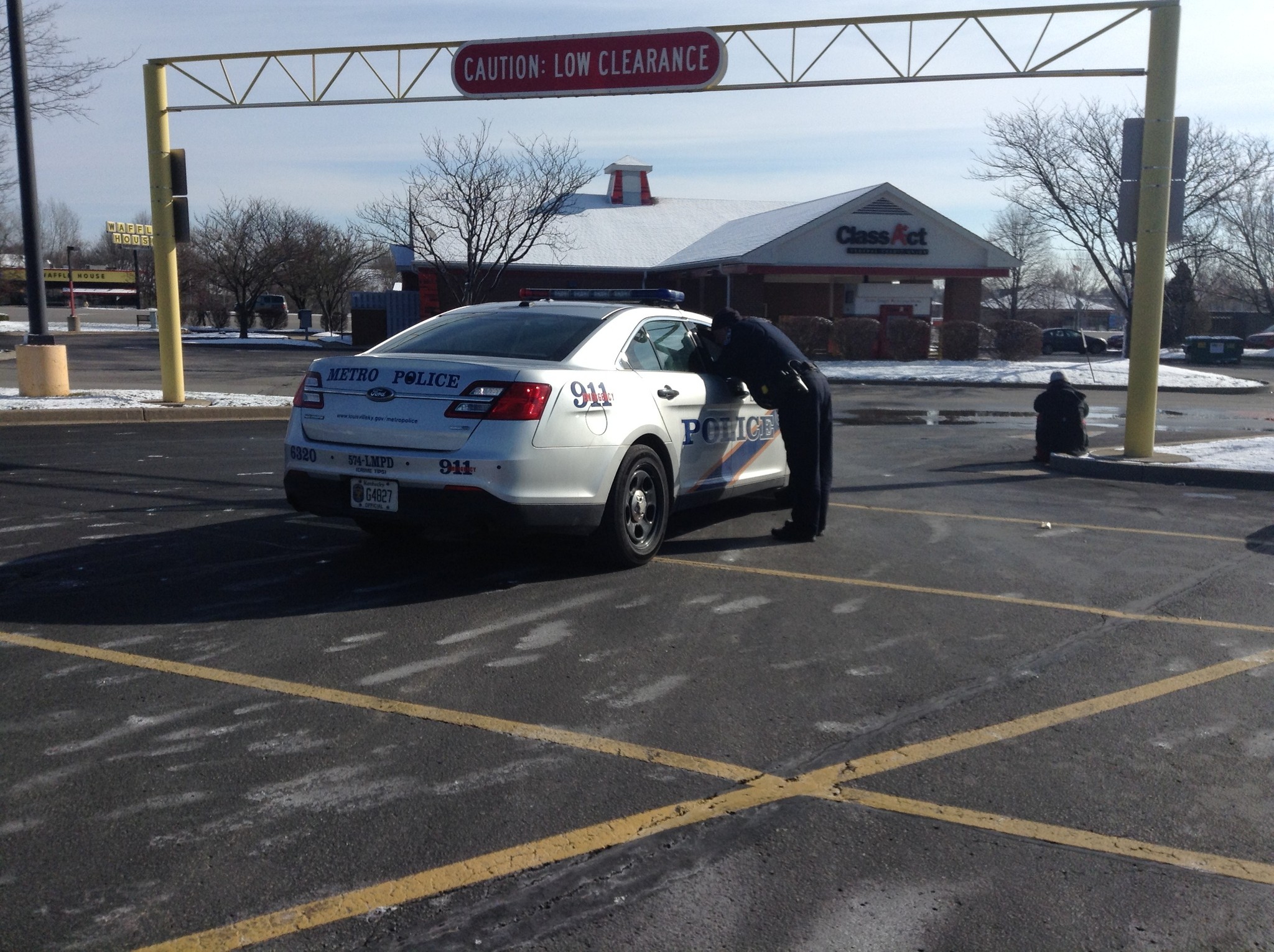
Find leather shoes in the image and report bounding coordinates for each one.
[771,527,816,541]
[785,519,823,536]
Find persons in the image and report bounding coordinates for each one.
[702,304,835,544]
[1032,369,1091,463]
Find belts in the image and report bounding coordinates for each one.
[773,362,818,380]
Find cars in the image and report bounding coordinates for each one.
[1040,328,1108,355]
[1246,324,1274,348]
[234,294,289,315]
[282,287,794,568]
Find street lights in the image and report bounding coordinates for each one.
[67,246,80,316]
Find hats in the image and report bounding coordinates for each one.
[1050,370,1068,381]
[711,307,742,332]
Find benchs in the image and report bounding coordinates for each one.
[136,315,158,327]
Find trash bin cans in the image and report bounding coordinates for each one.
[350,309,387,345]
